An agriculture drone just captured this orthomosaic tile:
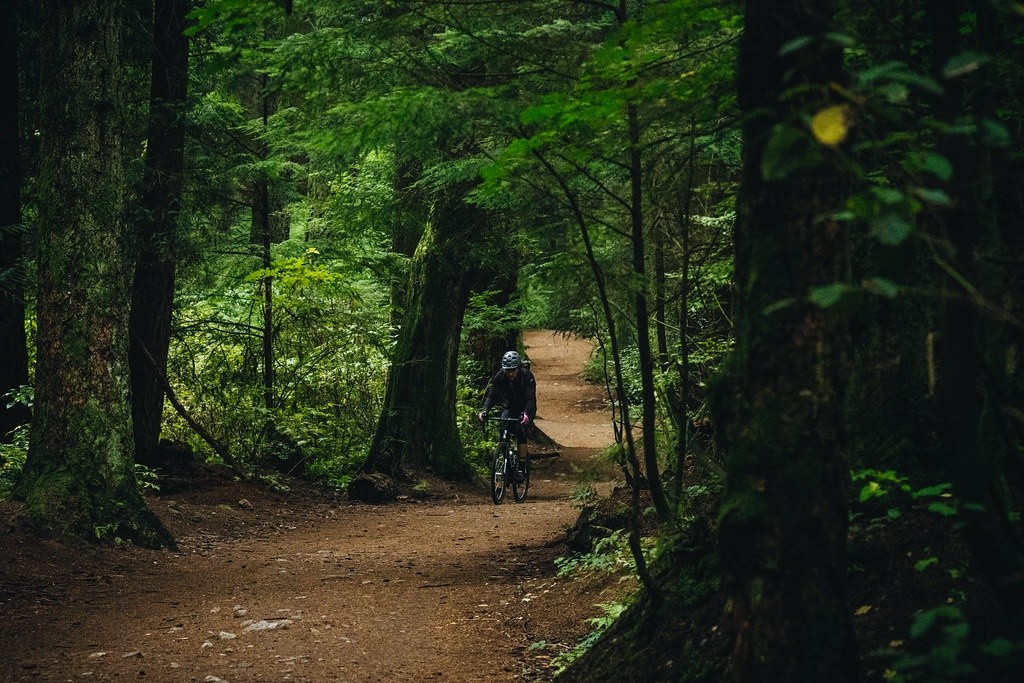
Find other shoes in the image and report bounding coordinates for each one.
[516,468,526,483]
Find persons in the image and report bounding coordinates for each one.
[479,351,537,483]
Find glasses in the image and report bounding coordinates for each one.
[505,369,517,372]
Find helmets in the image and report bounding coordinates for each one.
[501,351,520,369]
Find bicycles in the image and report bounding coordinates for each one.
[476,411,530,505]
